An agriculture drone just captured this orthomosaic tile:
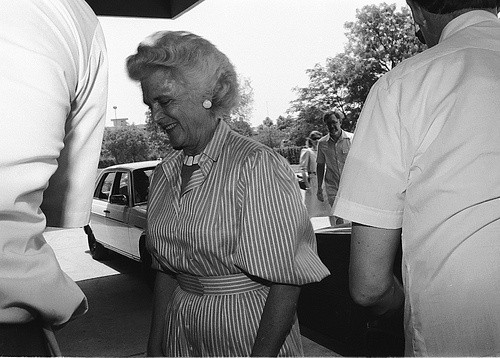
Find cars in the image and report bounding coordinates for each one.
[84,161,161,263]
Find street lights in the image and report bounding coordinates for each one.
[113,106,117,130]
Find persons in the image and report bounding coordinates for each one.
[299,111,355,226]
[123,30,330,358]
[0,0,109,357]
[134,171,151,203]
[331,0,500,358]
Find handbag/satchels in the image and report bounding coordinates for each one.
[298,178,310,189]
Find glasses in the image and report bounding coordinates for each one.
[311,137,321,141]
[413,20,426,44]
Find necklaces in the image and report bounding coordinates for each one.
[182,153,201,166]
